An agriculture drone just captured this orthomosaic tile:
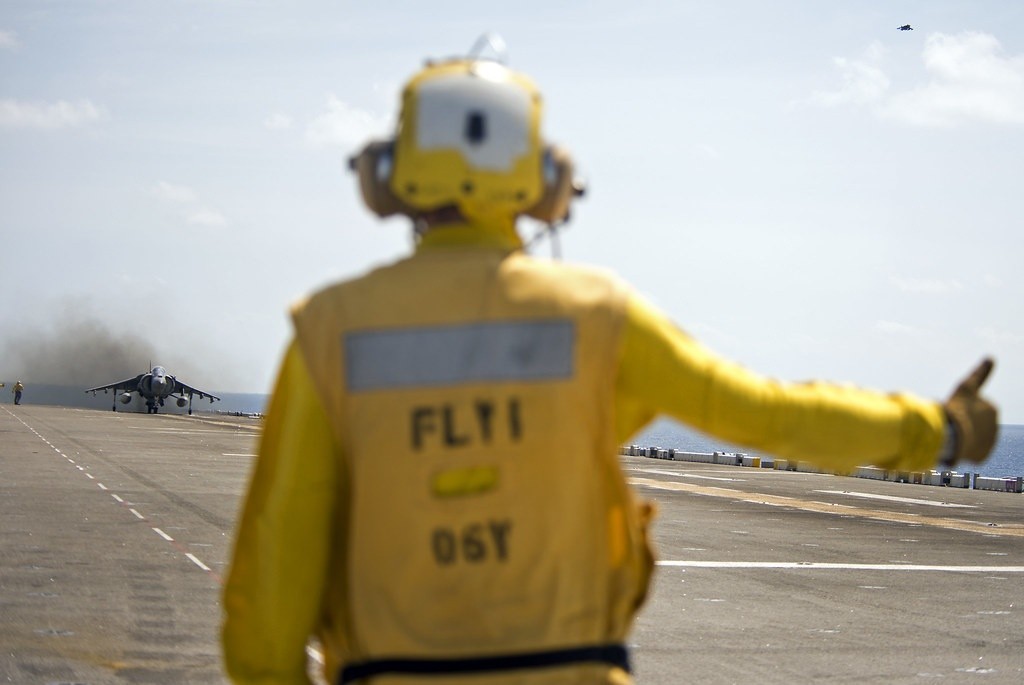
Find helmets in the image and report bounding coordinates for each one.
[357,59,574,230]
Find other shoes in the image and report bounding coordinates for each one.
[15,403,21,405]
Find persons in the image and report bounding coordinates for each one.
[12,381,24,405]
[223,30,998,685]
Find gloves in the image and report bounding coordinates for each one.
[944,358,1002,468]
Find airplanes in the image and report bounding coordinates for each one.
[83,359,220,416]
